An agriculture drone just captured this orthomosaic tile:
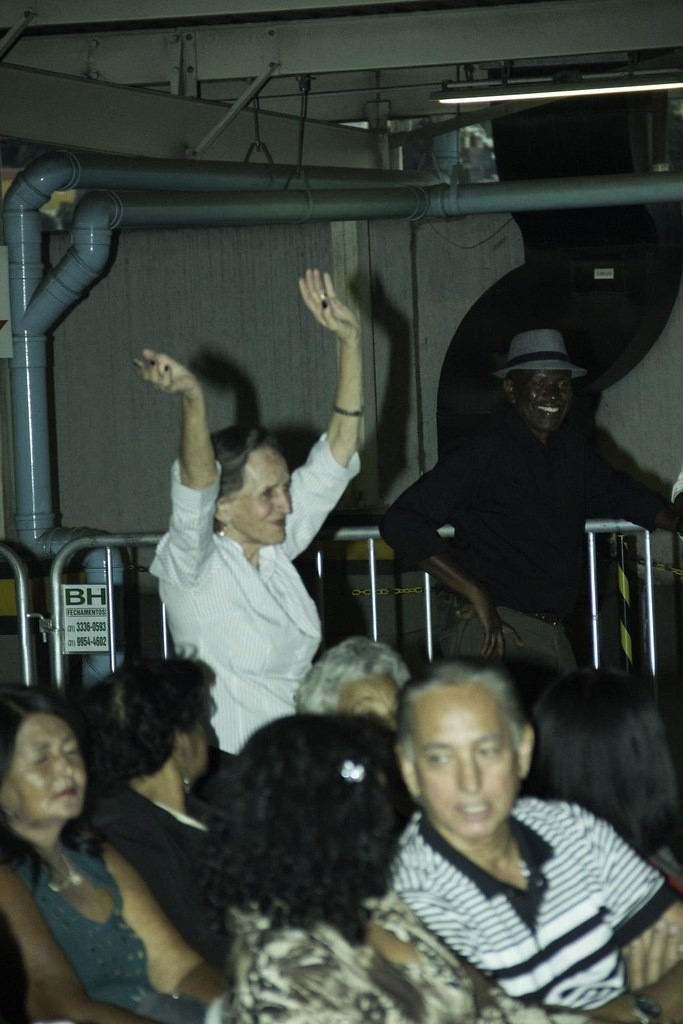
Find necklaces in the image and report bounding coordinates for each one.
[44,850,81,891]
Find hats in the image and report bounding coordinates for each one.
[491,328,587,379]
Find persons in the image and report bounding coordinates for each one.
[133,268,366,761]
[379,329,683,702]
[0,636,683,1024]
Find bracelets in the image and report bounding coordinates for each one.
[331,403,361,417]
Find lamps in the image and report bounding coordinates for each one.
[427,68,683,105]
